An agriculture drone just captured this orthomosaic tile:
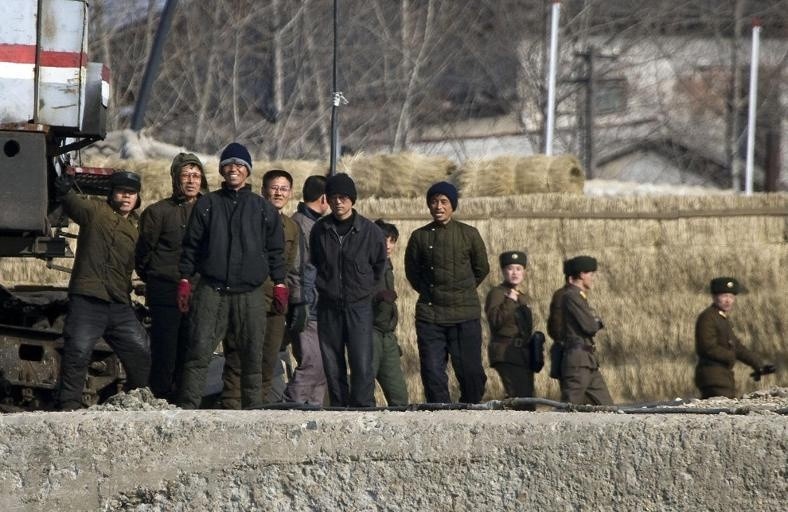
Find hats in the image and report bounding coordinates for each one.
[499,251,527,269]
[107,170,142,211]
[710,277,739,295]
[171,152,209,198]
[218,142,253,177]
[324,173,358,205]
[562,255,597,273]
[425,182,458,212]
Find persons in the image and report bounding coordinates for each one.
[374,218,408,407]
[136,152,209,406]
[405,181,490,404]
[216,170,300,409]
[310,172,387,408]
[695,277,765,399]
[51,171,151,411]
[562,257,614,407]
[485,252,545,411]
[284,175,330,405]
[547,258,571,392]
[177,142,290,409]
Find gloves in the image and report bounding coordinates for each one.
[53,173,74,196]
[749,364,777,382]
[175,281,192,313]
[376,289,398,306]
[272,286,289,315]
[292,304,311,333]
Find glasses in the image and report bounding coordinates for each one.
[177,172,201,179]
[225,163,246,168]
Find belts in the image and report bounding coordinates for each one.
[698,358,734,371]
[490,335,531,348]
[554,339,597,354]
